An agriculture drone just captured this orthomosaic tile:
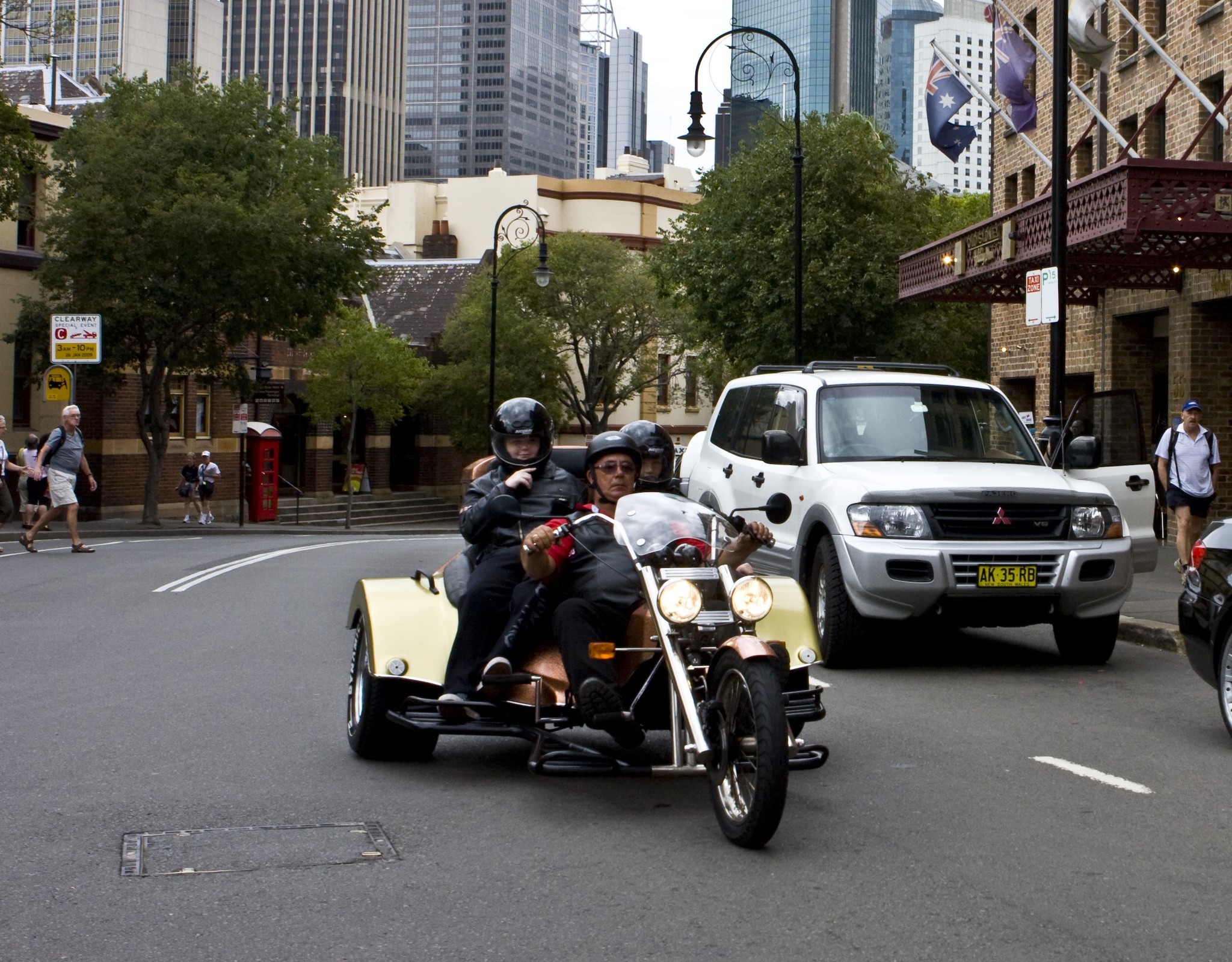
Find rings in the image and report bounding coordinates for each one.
[532,534,538,539]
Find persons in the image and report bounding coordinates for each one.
[983,431,1060,465]
[520,421,790,730]
[19,405,97,552]
[175,451,221,525]
[1155,400,1220,586]
[1072,420,1086,436]
[437,397,589,720]
[0,415,35,553]
[16,433,52,531]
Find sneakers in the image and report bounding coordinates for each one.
[183,515,190,523]
[198,519,207,524]
[206,516,214,524]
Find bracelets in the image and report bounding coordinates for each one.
[211,473,212,477]
[87,473,92,477]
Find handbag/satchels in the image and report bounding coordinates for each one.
[18,475,29,490]
[178,483,191,497]
[202,480,214,494]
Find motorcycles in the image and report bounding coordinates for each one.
[344,489,830,850]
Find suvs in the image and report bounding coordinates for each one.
[672,359,1161,667]
[1176,518,1232,741]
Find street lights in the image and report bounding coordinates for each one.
[678,25,806,365]
[486,202,555,458]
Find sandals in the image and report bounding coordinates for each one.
[19,534,38,552]
[71,543,95,553]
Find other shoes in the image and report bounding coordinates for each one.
[437,692,481,723]
[578,677,646,749]
[22,524,33,529]
[1181,573,1187,586]
[1173,558,1186,574]
[476,656,512,701]
[43,525,52,531]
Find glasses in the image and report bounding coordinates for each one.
[66,414,82,419]
[0,426,7,429]
[594,459,638,475]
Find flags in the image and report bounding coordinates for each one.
[994,5,1038,138]
[1068,0,1117,75]
[924,49,978,164]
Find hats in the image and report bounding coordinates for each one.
[201,450,211,457]
[1182,400,1203,412]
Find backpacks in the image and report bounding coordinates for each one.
[37,425,84,466]
[1151,426,1213,489]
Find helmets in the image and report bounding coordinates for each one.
[489,397,554,480]
[584,430,644,505]
[619,420,675,492]
